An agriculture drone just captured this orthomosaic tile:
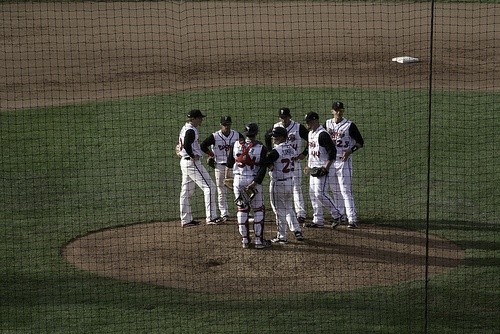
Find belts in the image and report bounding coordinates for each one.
[185,157,191,160]
[276,177,293,182]
[219,163,226,165]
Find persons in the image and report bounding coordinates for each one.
[175,109,226,227]
[265,128,304,243]
[201,115,255,222]
[227,123,272,249]
[303,112,344,229]
[266,106,309,221]
[321,101,364,228]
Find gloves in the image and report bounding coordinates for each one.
[341,146,352,162]
[311,166,329,179]
[207,156,217,168]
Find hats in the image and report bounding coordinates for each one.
[245,123,258,136]
[271,127,288,137]
[332,102,345,110]
[220,116,232,123]
[278,107,292,119]
[187,109,206,119]
[302,112,320,122]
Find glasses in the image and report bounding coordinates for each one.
[335,109,344,113]
[222,123,231,126]
[278,116,288,120]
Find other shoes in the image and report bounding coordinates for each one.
[294,231,304,241]
[330,214,347,225]
[223,215,229,220]
[297,217,306,223]
[242,242,250,249]
[302,221,324,228]
[184,219,203,228]
[254,239,272,248]
[207,216,226,224]
[268,237,288,244]
[347,222,358,227]
